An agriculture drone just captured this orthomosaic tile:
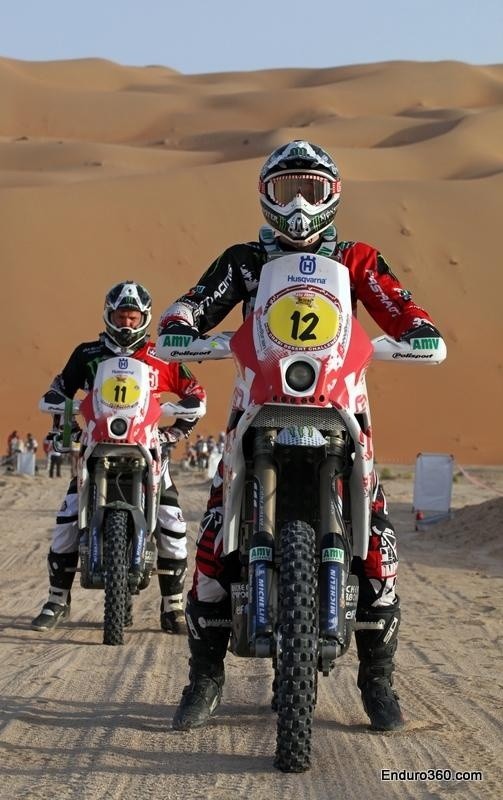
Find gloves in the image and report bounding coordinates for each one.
[159,427,185,448]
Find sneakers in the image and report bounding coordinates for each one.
[160,610,188,634]
[357,678,404,731]
[31,601,71,632]
[172,671,225,731]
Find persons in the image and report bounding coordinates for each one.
[8,422,226,478]
[159,139,446,731]
[8,283,205,634]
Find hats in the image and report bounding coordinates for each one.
[257,139,341,243]
[103,280,152,348]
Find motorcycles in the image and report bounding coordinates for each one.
[157,251,450,775]
[36,354,205,648]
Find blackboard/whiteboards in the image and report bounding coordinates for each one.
[412,453,454,514]
